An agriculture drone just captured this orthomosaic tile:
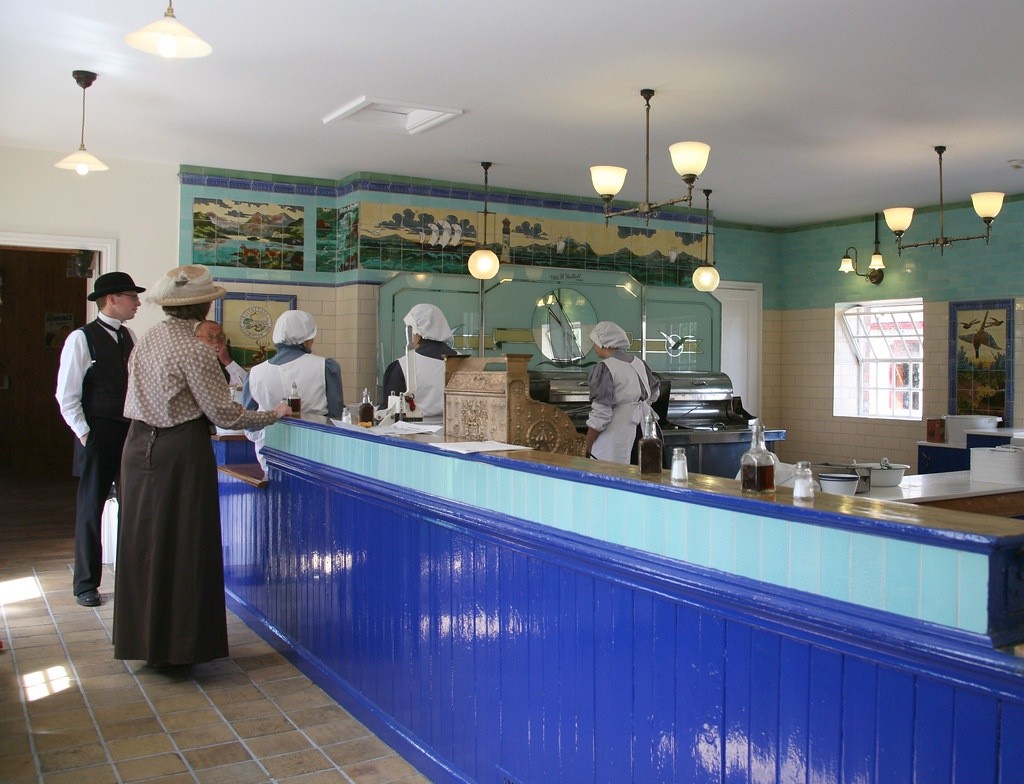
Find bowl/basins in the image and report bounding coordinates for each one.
[819,474,860,496]
[854,462,910,487]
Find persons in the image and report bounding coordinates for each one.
[194,320,249,404]
[376,304,463,417]
[55,271,146,606]
[585,321,660,466]
[240,310,343,475]
[112,264,292,674]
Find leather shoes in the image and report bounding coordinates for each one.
[75,588,102,606]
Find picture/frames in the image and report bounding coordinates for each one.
[946,299,1015,428]
[215,290,297,371]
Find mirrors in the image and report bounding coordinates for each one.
[532,289,597,362]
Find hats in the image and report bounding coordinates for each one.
[88,272,146,301]
[146,264,228,307]
[270,309,318,346]
[404,303,454,343]
[590,321,631,348]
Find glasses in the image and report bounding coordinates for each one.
[194,332,225,342]
[112,292,139,299]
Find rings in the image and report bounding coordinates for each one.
[217,348,219,351]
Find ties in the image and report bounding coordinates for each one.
[113,330,125,352]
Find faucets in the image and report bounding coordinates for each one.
[712,422,727,430]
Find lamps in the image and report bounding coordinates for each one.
[467,164,500,279]
[123,0,213,60]
[838,247,884,285]
[883,146,1005,258]
[589,94,712,229]
[867,213,886,271]
[54,69,109,176]
[692,190,720,293]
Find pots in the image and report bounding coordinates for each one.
[944,414,1004,446]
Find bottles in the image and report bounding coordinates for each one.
[342,406,353,425]
[792,461,814,500]
[670,447,689,483]
[740,418,777,493]
[280,397,287,405]
[287,383,302,417]
[638,416,662,473]
[358,390,374,428]
